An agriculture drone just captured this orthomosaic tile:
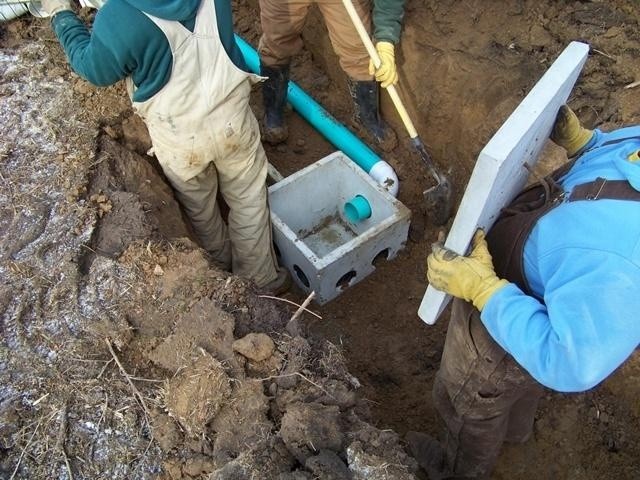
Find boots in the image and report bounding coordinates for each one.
[507,378,542,445]
[259,59,290,144]
[346,74,398,153]
[405,372,510,480]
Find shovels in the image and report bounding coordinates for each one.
[341,0,456,227]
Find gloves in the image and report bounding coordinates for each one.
[547,102,596,161]
[368,38,400,89]
[426,227,509,316]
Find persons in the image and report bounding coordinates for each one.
[256,1,405,153]
[403,102,640,478]
[35,0,293,299]
